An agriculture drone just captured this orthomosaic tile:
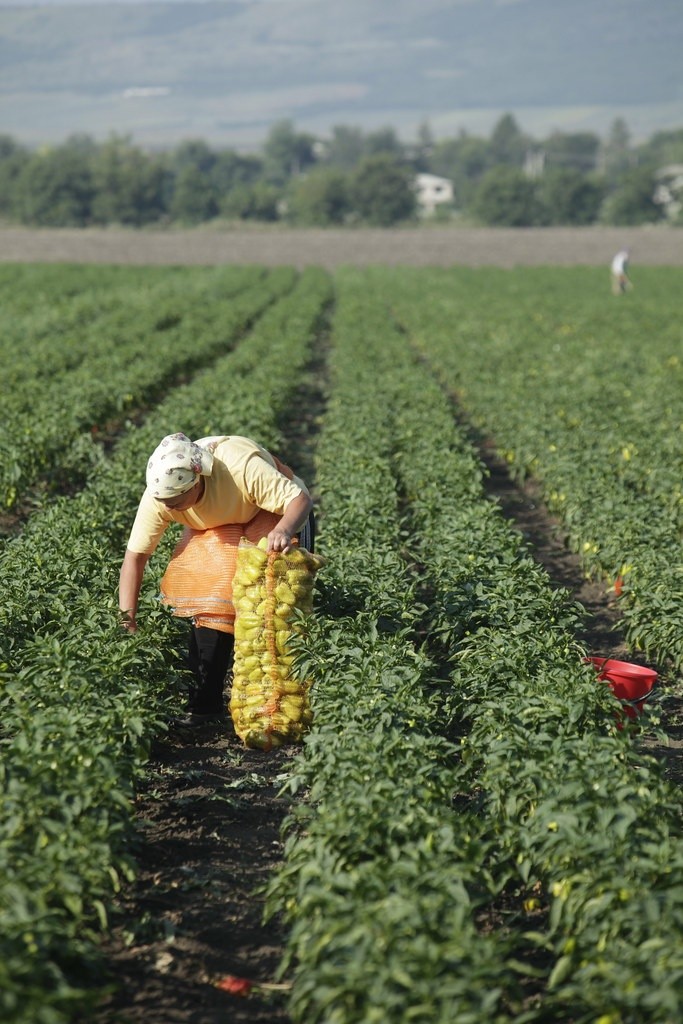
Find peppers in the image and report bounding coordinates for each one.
[230,536,326,748]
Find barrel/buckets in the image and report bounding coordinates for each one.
[583,657,658,730]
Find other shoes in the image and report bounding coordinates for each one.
[176,709,227,728]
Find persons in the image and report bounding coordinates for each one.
[611,248,635,297]
[118,432,318,720]
